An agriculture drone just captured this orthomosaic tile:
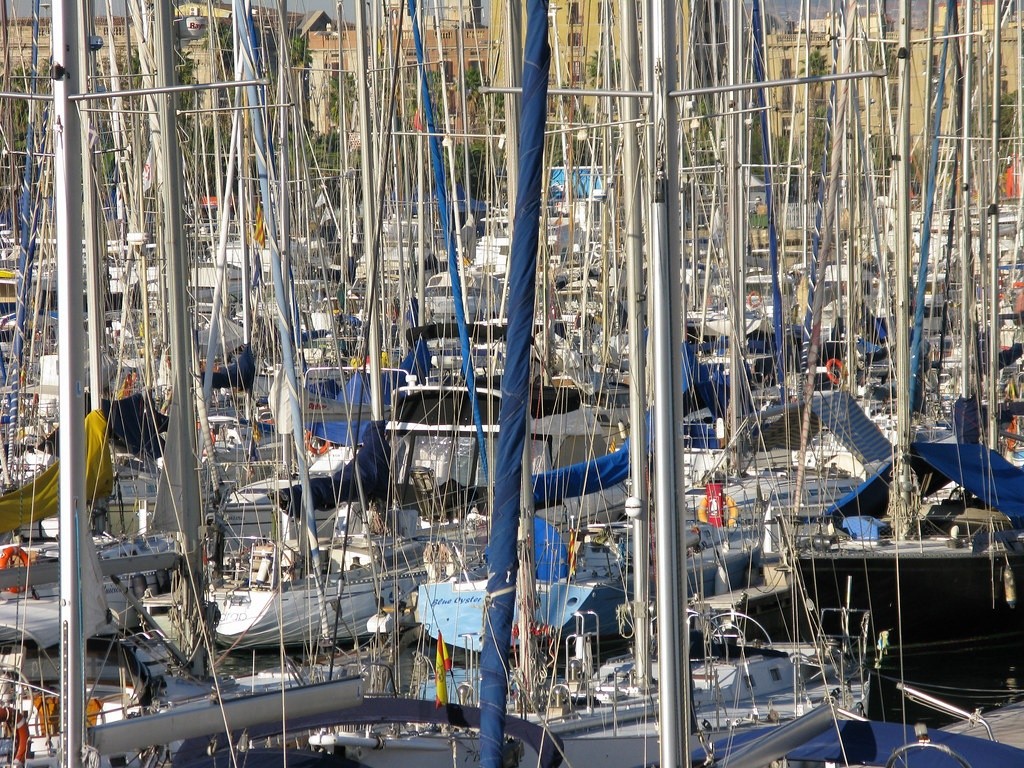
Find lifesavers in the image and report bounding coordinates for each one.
[258,409,274,424]
[826,358,844,383]
[698,493,738,526]
[197,422,215,443]
[387,302,398,321]
[0,546,32,593]
[306,432,330,454]
[748,291,762,307]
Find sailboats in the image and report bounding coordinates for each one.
[0,0,1024,768]
